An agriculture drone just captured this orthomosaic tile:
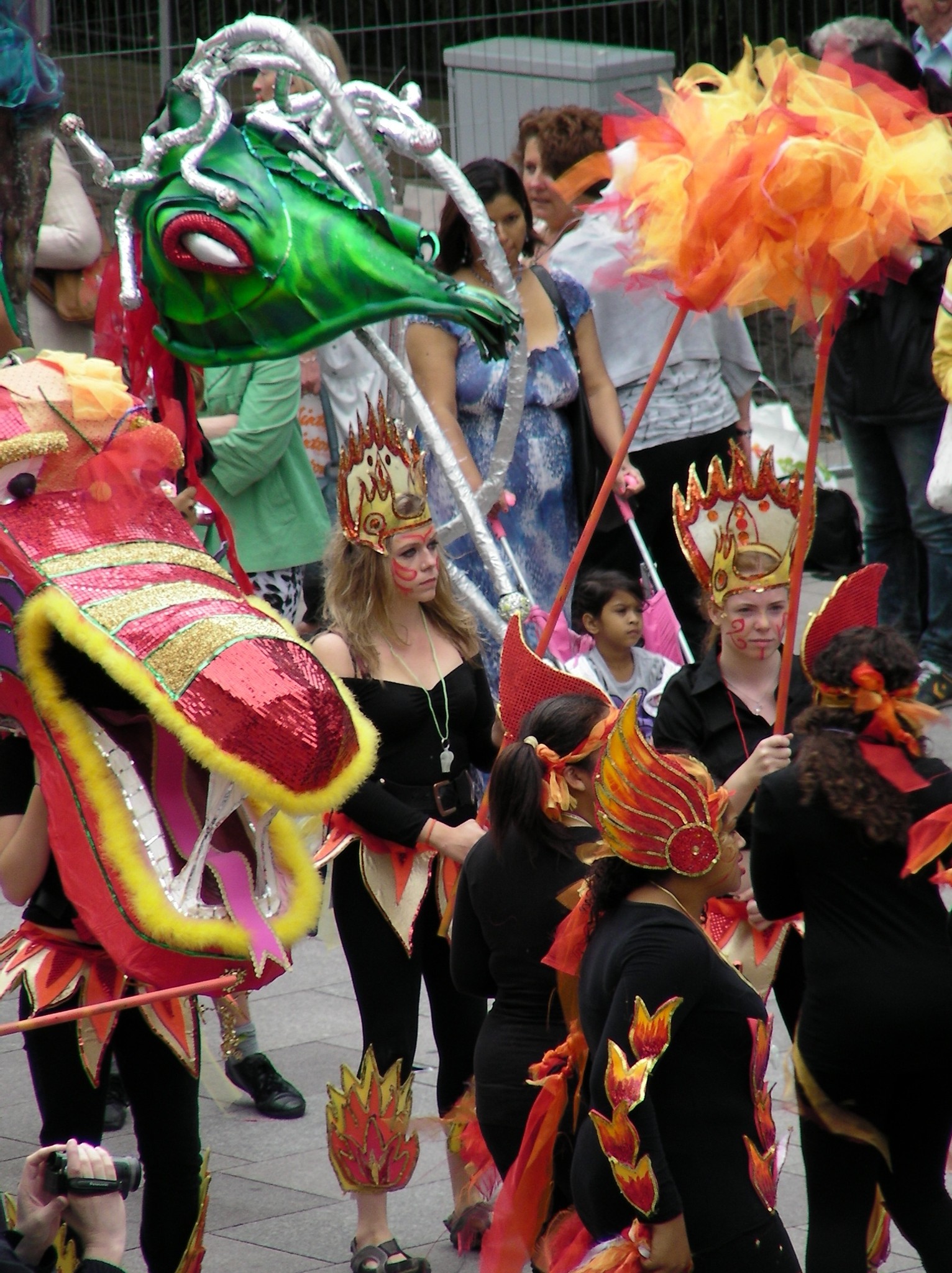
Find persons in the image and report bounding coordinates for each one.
[563,576,681,745]
[398,156,647,808]
[59,12,532,646]
[902,0,952,86]
[512,108,623,274]
[537,105,762,662]
[652,439,823,1273]
[925,258,952,513]
[312,389,505,1273]
[0,346,380,1273]
[448,611,618,1273]
[807,17,952,711]
[0,1138,142,1273]
[26,133,105,359]
[253,21,351,525]
[479,690,805,1273]
[751,562,952,1273]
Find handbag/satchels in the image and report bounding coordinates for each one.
[31,226,106,325]
[530,263,595,525]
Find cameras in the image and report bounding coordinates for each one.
[46,1152,141,1199]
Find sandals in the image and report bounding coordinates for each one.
[349,1233,432,1273]
[443,1200,491,1249]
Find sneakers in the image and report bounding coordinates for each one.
[224,1051,306,1120]
[103,1074,127,1132]
[913,661,952,710]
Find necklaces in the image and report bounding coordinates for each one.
[388,602,455,773]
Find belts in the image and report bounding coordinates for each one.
[404,770,478,816]
